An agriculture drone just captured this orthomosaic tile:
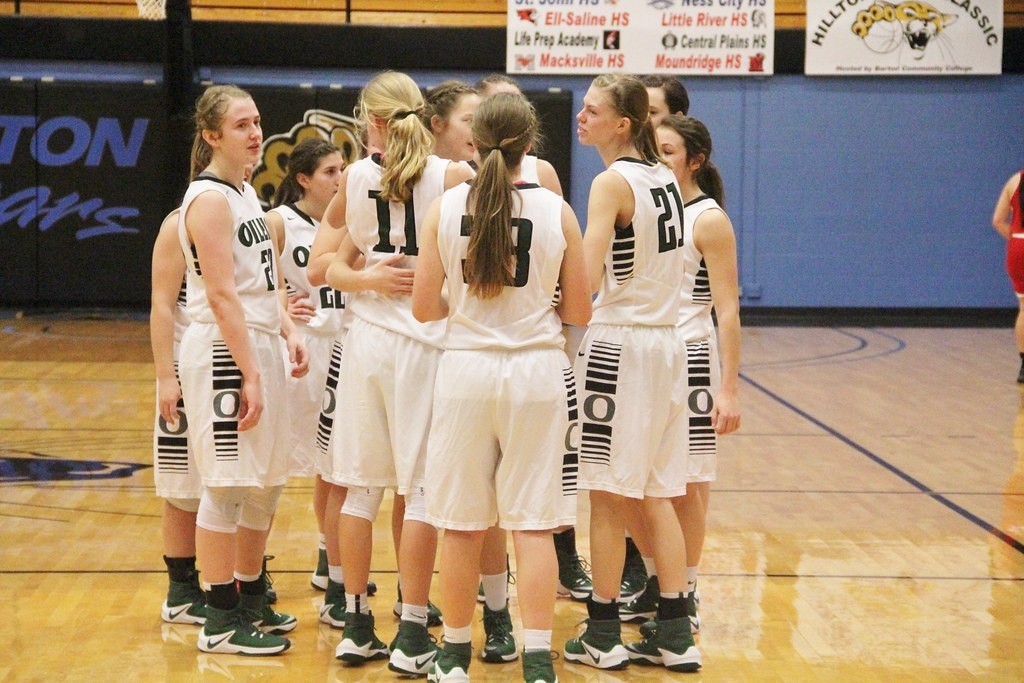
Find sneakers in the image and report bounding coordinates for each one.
[160,570,208,625]
[477,552,703,683]
[197,608,291,655]
[1017,370,1024,383]
[262,568,276,601]
[309,544,474,683]
[247,604,298,634]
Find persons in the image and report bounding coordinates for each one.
[992,168,1024,384]
[150,70,741,683]
